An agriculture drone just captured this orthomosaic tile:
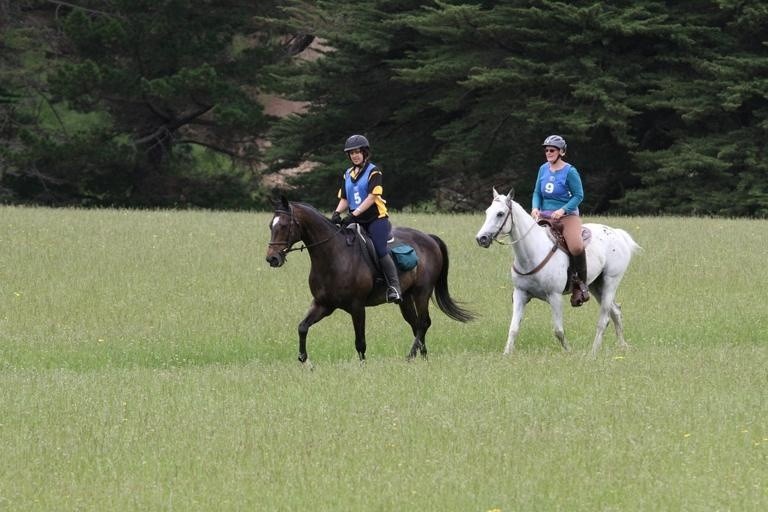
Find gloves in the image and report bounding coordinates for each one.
[341,213,355,228]
[331,211,340,223]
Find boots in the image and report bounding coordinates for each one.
[569,249,587,307]
[378,253,403,304]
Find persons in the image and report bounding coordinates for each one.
[529,135,591,308]
[329,133,403,307]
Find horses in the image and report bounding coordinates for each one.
[267,188,481,372]
[476,186,646,362]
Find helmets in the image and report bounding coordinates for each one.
[542,134,567,151]
[344,135,369,152]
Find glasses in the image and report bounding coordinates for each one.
[545,149,555,152]
